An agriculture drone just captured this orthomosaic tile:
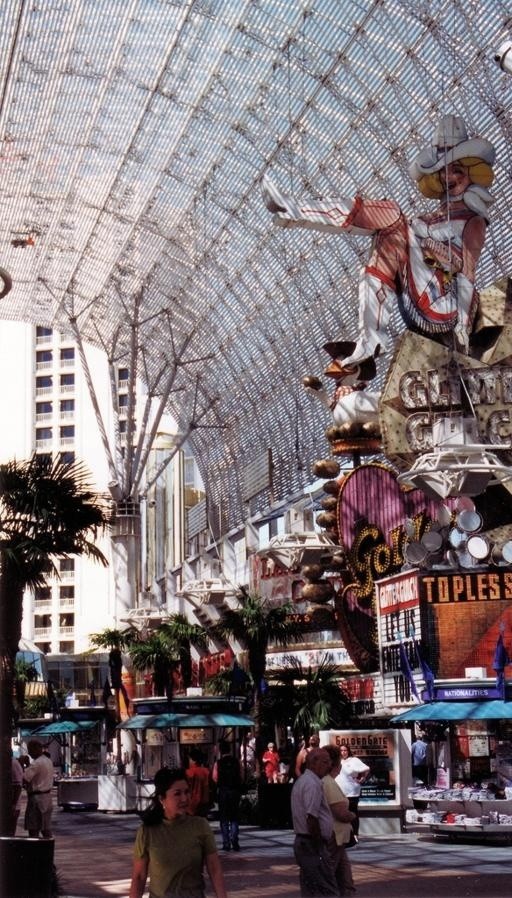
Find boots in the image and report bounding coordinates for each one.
[258,173,364,234]
[335,269,391,365]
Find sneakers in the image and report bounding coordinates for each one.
[222,839,240,854]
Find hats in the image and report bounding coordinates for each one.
[277,762,290,776]
[416,111,497,173]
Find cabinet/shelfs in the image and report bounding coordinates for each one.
[402,795,512,840]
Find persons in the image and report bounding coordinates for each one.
[180,733,373,852]
[10,748,24,836]
[411,737,428,783]
[129,765,229,897]
[291,748,350,898]
[16,750,51,838]
[322,743,359,898]
[18,741,54,838]
